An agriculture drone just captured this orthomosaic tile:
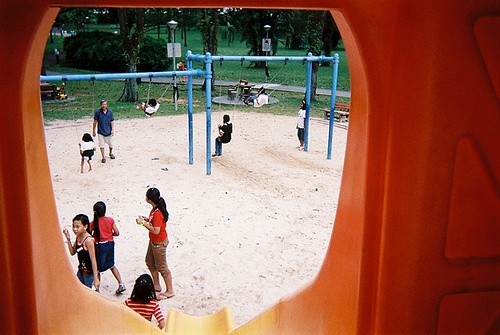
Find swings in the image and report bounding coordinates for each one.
[62,82,94,159]
[144,78,173,117]
[245,64,304,106]
[217,61,243,144]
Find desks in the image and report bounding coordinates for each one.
[323,102,350,122]
[239,82,255,100]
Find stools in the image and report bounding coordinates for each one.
[228,88,238,100]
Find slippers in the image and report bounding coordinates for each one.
[154,286,161,292]
[157,294,174,300]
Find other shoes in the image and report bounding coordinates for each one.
[110,153,115,159]
[212,154,221,157]
[102,159,105,163]
[296,145,303,150]
[116,285,125,293]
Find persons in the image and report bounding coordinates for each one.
[79,133,96,173]
[60,83,67,99]
[61,31,64,36]
[137,99,160,116]
[135,188,174,300]
[56,50,60,68]
[64,214,99,290]
[212,115,232,156]
[245,86,269,107]
[89,202,126,293]
[179,60,185,83]
[296,100,307,148]
[93,99,115,163]
[123,274,165,330]
[71,30,75,36]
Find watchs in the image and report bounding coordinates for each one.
[140,220,146,226]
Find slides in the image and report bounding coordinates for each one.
[0,0,500,335]
